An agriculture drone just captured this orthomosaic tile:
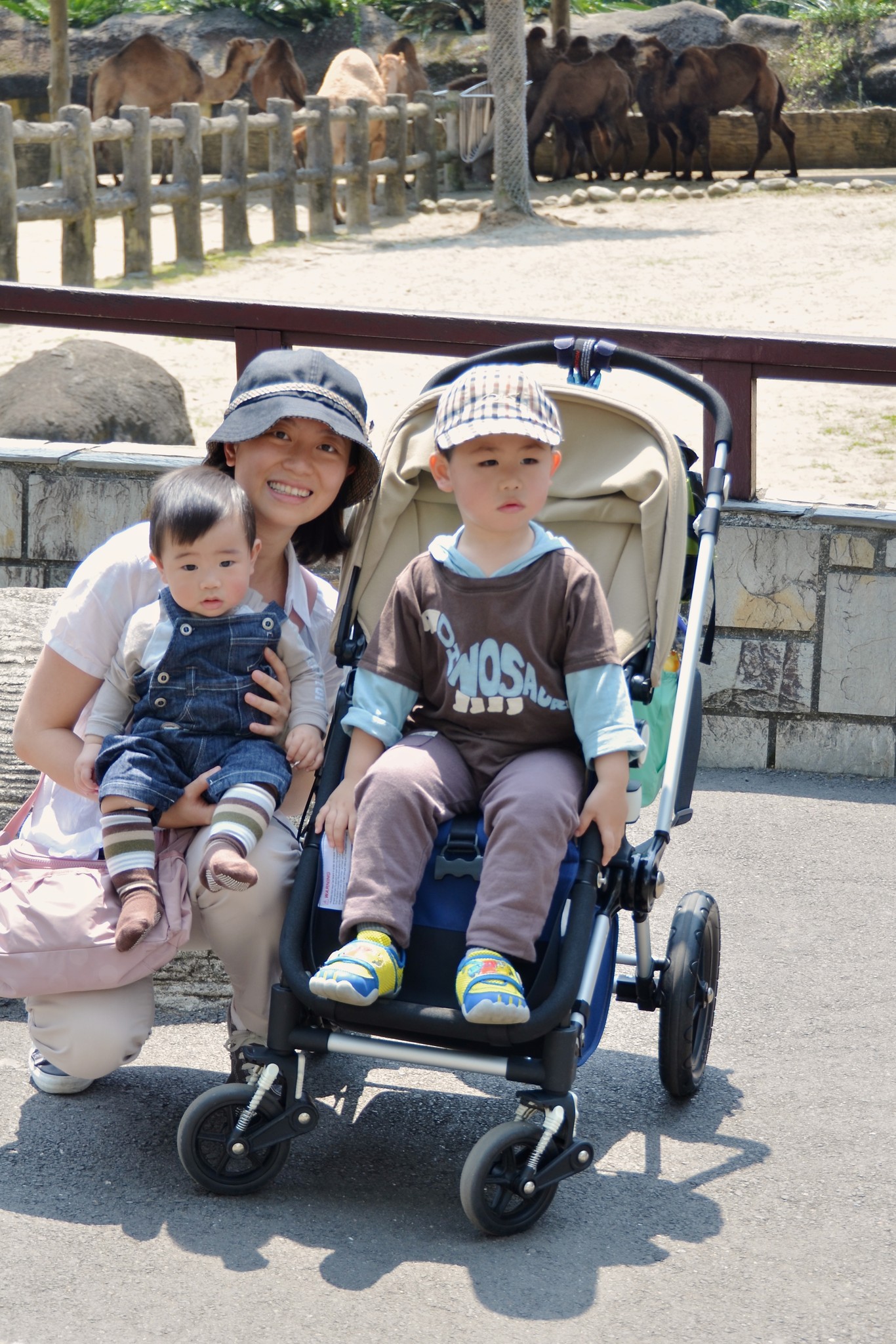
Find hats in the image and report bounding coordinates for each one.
[433,363,563,450]
[205,348,382,509]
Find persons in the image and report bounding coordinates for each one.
[70,465,326,956]
[309,364,652,1027]
[7,345,382,1098]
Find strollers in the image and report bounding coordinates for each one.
[175,333,738,1240]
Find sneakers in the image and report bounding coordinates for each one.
[455,950,531,1025]
[224,1000,287,1136]
[28,1045,97,1095]
[309,929,407,1006]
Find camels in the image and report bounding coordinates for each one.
[89,26,797,226]
